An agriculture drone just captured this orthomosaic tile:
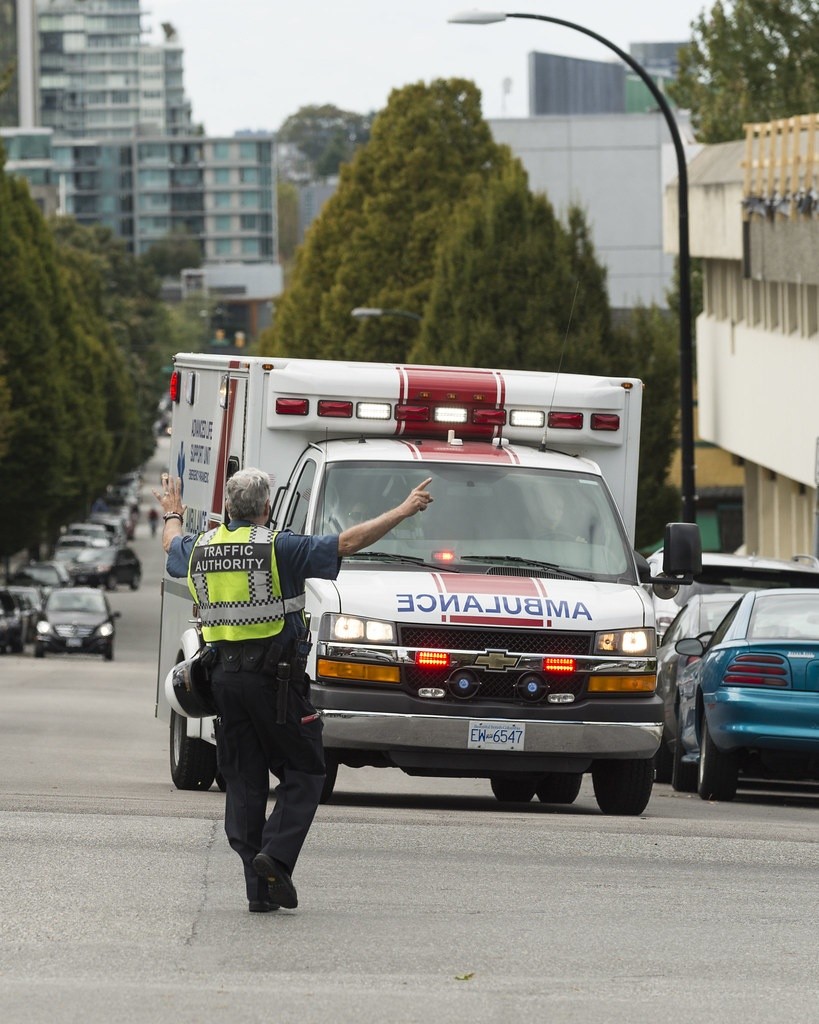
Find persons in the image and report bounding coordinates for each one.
[344,498,372,528]
[153,467,434,914]
[510,493,581,541]
[149,508,159,535]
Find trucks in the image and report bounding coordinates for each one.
[162,350,664,818]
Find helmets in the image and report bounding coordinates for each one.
[164,658,219,719]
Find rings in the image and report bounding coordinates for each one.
[164,492,170,495]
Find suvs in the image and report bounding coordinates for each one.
[645,547,819,652]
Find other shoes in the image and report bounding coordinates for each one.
[248,901,281,912]
[251,851,300,909]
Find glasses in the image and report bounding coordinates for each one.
[347,511,372,522]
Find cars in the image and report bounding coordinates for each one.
[0,469,152,659]
[669,588,819,801]
[652,591,744,794]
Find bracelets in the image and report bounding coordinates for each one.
[163,511,183,525]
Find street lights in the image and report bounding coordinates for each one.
[445,7,699,571]
